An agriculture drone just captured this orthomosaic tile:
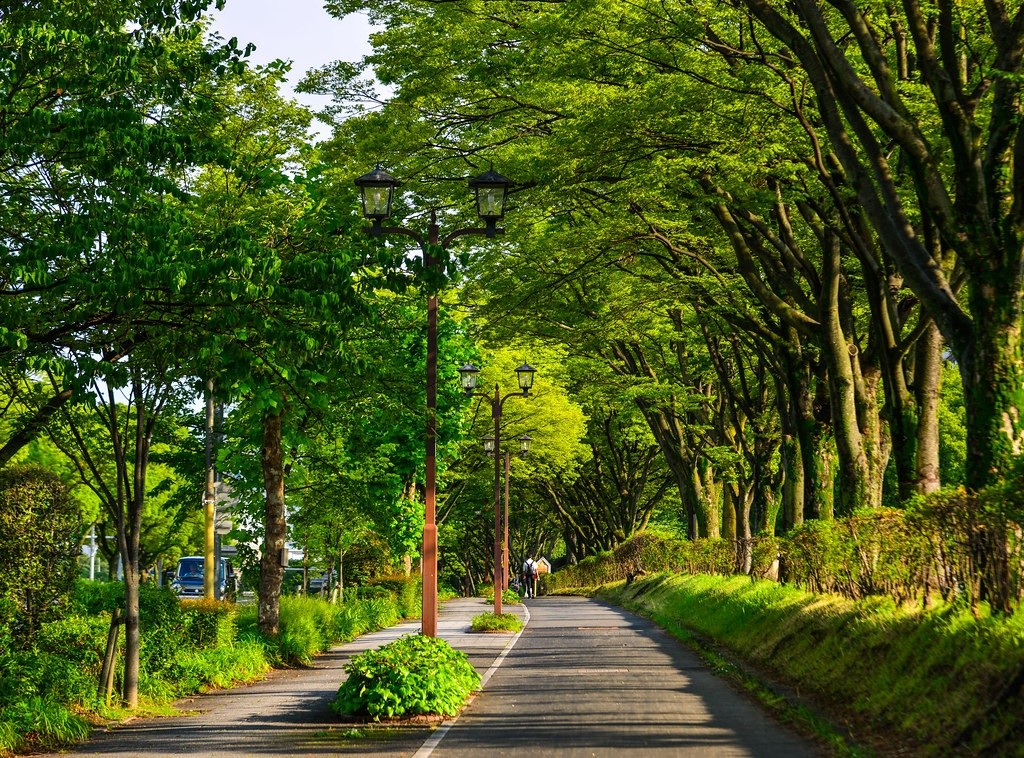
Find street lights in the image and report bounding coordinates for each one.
[353,161,517,642]
[456,356,538,615]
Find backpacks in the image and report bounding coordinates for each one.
[524,560,535,576]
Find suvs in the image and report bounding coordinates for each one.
[165,556,237,605]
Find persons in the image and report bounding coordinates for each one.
[512,574,522,597]
[523,554,539,599]
[184,563,203,579]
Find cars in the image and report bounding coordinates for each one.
[309,578,329,595]
